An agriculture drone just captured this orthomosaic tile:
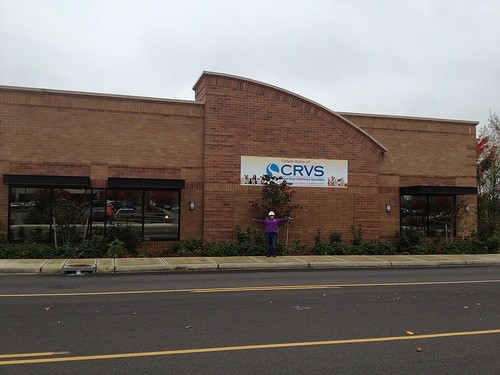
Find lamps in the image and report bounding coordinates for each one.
[465,205,470,213]
[386,204,391,212]
[189,201,194,210]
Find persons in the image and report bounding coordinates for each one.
[106,203,113,224]
[252,212,292,258]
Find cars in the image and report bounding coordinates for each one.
[10,198,173,224]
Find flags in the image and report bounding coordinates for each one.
[476,136,489,155]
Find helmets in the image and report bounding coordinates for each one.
[268,211,275,216]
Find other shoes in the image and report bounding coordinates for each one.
[273,254,276,257]
[267,254,270,257]
